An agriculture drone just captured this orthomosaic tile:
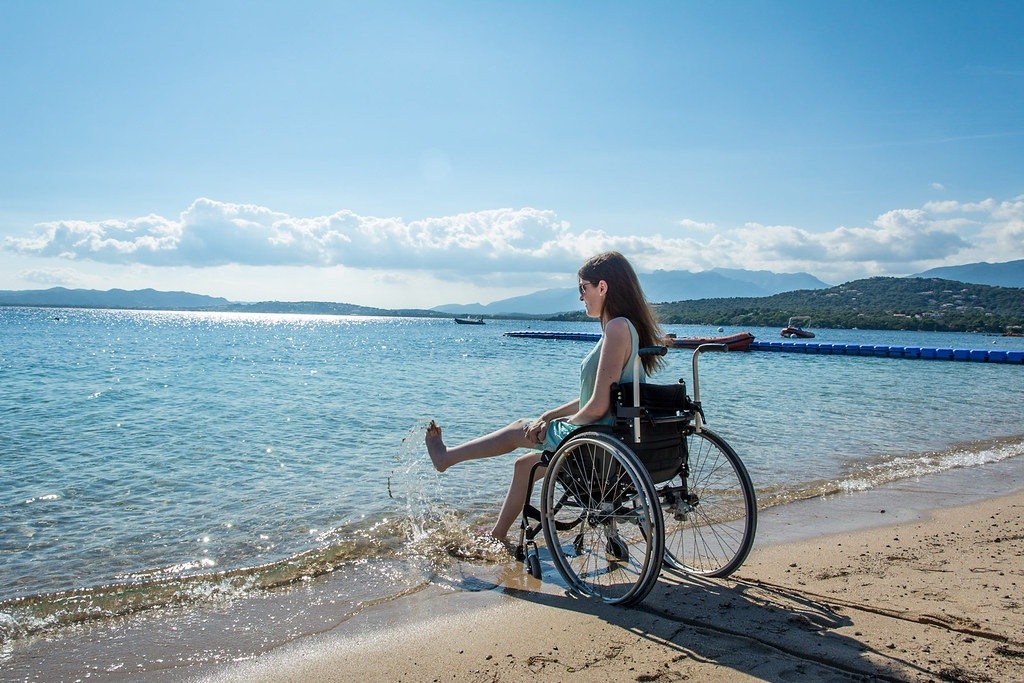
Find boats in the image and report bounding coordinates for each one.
[454,317,485,324]
[781,326,816,339]
[669,331,752,350]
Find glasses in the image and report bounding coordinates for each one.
[579,282,599,294]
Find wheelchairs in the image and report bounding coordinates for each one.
[512,338,760,612]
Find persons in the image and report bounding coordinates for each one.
[481,315,483,322]
[425,251,669,539]
